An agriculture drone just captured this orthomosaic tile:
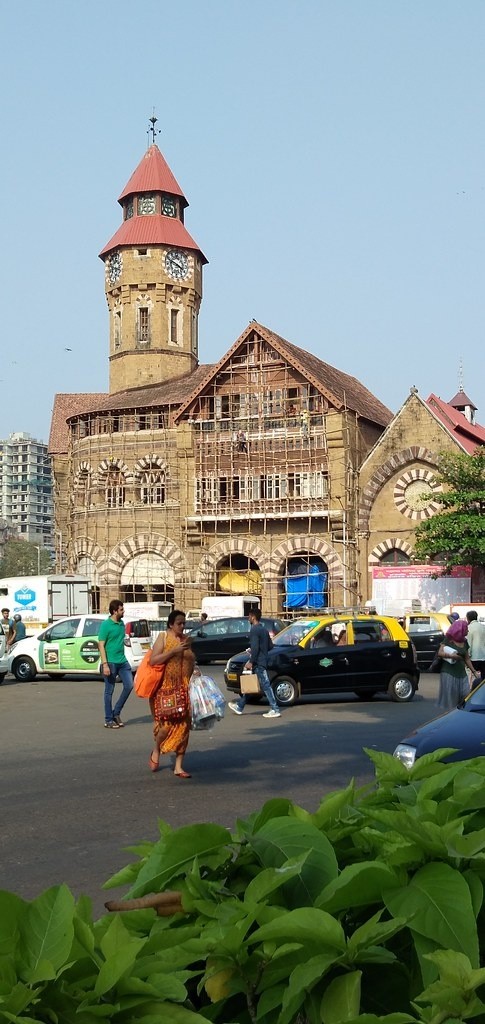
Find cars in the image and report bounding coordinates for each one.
[148,616,295,649]
[223,614,421,701]
[394,679,485,772]
[380,613,457,671]
[185,617,285,665]
[8,614,152,681]
[0,624,10,684]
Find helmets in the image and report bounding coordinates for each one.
[14,614,22,622]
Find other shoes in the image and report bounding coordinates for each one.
[114,717,124,727]
[104,721,119,729]
[228,702,243,715]
[262,709,282,718]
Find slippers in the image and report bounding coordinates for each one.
[175,770,192,778]
[150,750,160,771]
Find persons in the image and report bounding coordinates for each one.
[237,430,247,453]
[436,619,479,712]
[447,612,459,624]
[149,610,200,778]
[200,613,207,622]
[228,609,281,718]
[7,614,26,645]
[309,627,325,649]
[466,611,485,681]
[302,409,311,436]
[98,600,133,728]
[287,404,296,413]
[0,608,12,635]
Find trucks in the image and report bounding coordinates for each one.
[121,602,171,623]
[0,576,91,625]
[187,595,261,620]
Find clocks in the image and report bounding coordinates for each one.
[165,251,189,278]
[108,253,122,282]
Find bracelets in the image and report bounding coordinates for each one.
[103,662,107,664]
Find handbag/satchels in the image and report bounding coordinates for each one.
[134,630,166,698]
[154,684,188,720]
[240,667,261,693]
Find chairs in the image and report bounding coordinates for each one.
[324,631,334,647]
[336,630,356,646]
